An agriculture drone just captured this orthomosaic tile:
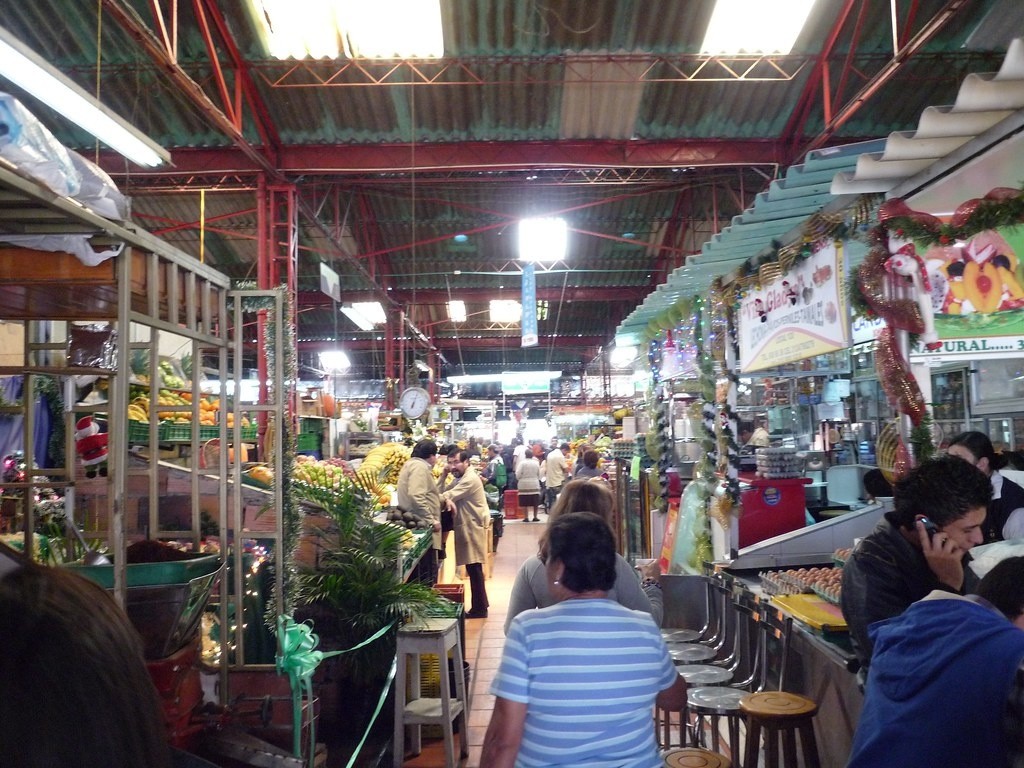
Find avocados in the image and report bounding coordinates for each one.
[386,504,427,531]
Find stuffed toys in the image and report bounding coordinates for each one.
[74,415,109,478]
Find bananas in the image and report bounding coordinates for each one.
[128,396,153,423]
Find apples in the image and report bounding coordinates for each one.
[293,453,391,512]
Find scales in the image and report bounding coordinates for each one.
[399,385,436,432]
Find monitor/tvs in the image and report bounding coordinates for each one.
[815,403,844,422]
[821,379,851,402]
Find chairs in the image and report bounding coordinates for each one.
[652,559,794,768]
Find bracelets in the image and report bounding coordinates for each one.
[640,576,663,590]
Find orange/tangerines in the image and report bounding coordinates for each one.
[157,390,247,428]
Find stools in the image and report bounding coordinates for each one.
[738,691,820,768]
[307,387,328,417]
[660,747,734,768]
[393,617,469,768]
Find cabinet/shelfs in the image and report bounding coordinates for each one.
[345,437,382,460]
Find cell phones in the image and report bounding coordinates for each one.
[912,517,947,549]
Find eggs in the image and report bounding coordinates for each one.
[768,548,854,599]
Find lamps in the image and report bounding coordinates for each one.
[0,25,178,170]
[340,302,374,331]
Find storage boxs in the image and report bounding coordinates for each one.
[402,525,433,579]
[503,490,525,519]
[93,412,324,461]
[432,583,464,602]
[406,649,453,738]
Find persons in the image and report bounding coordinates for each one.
[505,480,663,637]
[0,563,174,768]
[839,453,995,698]
[480,512,688,768]
[397,439,442,590]
[437,448,491,619]
[438,437,515,511]
[846,556,1024,768]
[948,431,1024,548]
[738,421,769,455]
[515,434,571,522]
[575,444,606,479]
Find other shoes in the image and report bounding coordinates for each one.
[523,518,529,522]
[464,608,488,618]
[533,519,540,521]
